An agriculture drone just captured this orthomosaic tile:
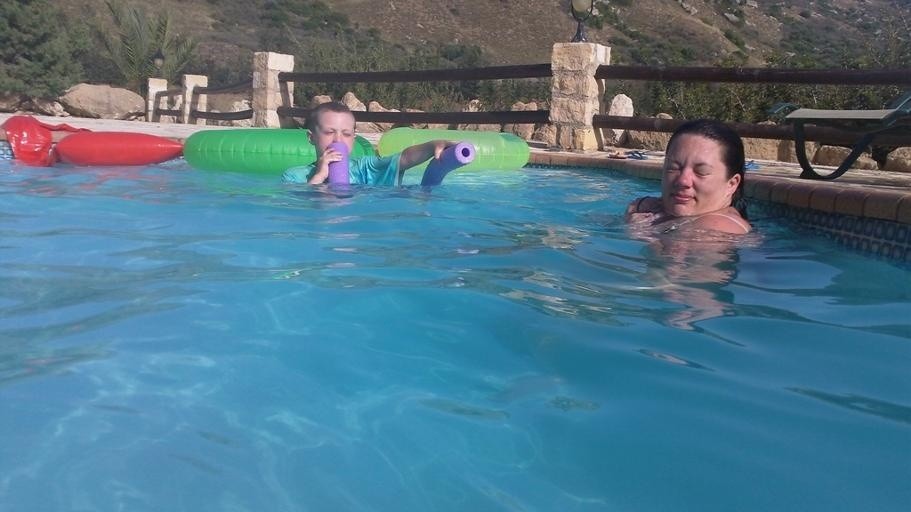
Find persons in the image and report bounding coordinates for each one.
[281,102,462,192]
[622,119,754,242]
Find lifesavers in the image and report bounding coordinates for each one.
[380,127,531,171]
[183,129,377,174]
[1,116,183,165]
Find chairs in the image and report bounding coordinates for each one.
[784,92,910,184]
